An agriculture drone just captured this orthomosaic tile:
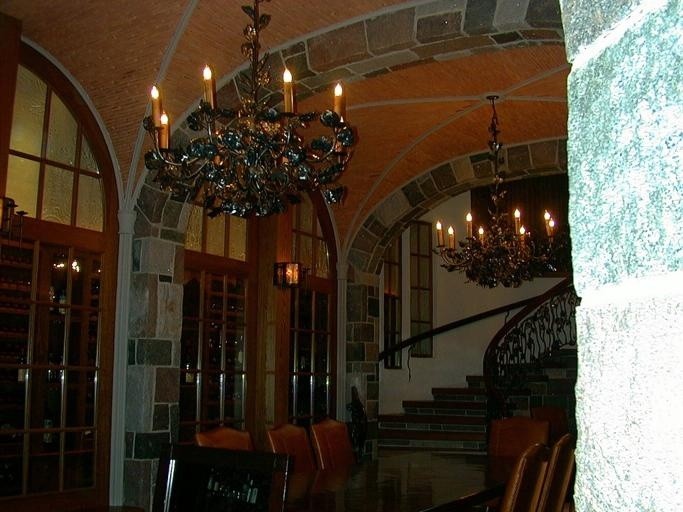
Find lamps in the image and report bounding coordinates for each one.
[273,259,303,289]
[428,91,571,290]
[135,0,355,216]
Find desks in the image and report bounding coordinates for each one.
[272,452,565,511]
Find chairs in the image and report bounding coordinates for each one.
[150,421,378,511]
[432,400,578,510]
